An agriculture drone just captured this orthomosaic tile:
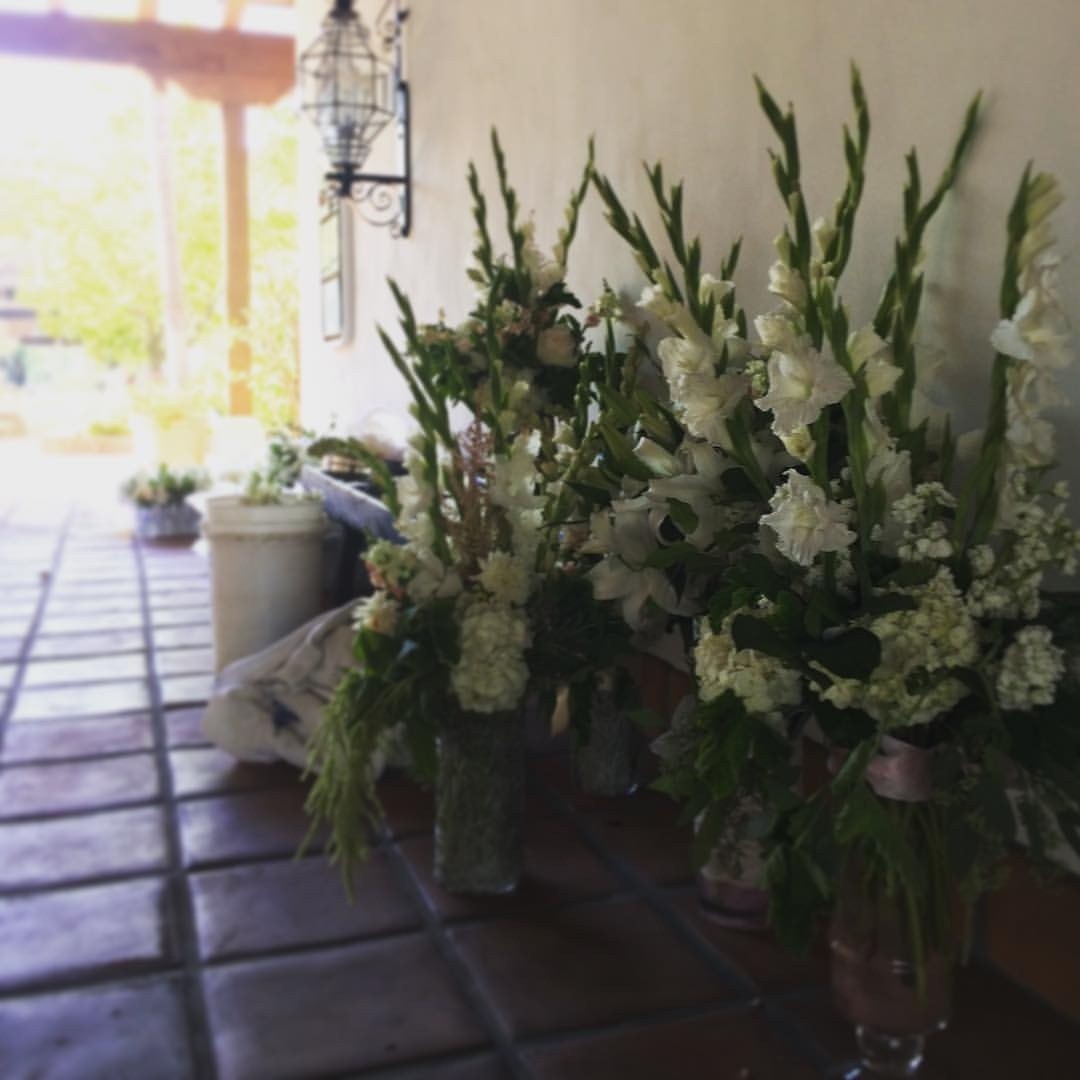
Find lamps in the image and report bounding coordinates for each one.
[296,0,414,240]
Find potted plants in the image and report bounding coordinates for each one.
[135,462,201,545]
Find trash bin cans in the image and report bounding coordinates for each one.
[205,499,333,671]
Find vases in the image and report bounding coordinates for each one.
[691,866,777,934]
[827,844,957,1037]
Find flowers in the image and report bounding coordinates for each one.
[239,57,1080,946]
